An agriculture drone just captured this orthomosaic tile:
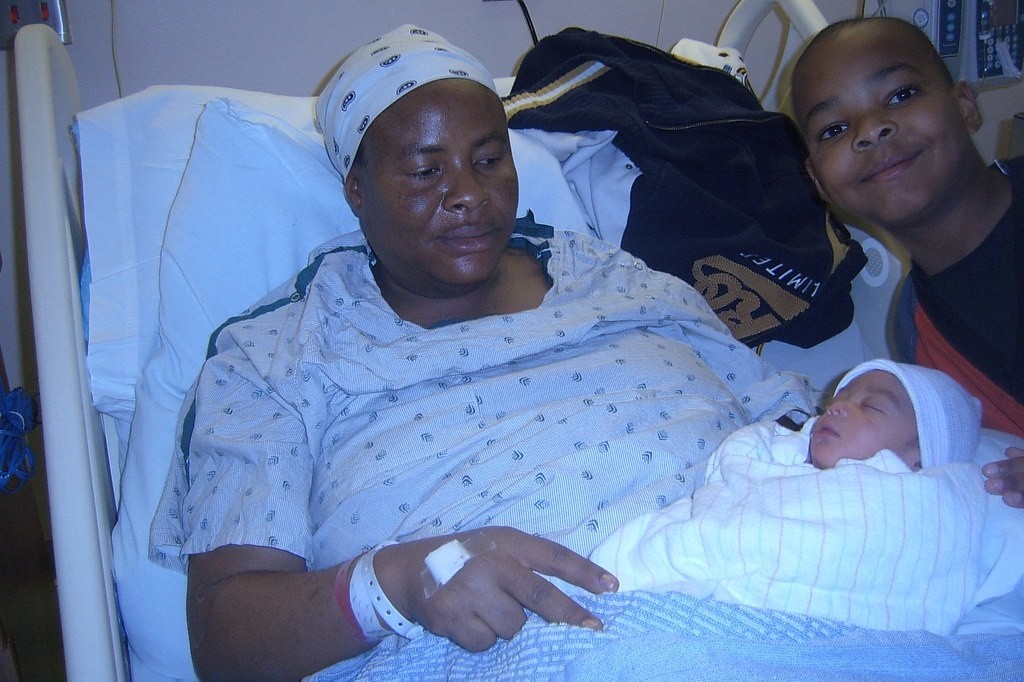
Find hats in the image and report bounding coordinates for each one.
[834,360,983,469]
[316,24,502,185]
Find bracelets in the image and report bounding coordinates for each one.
[339,540,427,643]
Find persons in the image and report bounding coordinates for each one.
[593,357,1024,633]
[789,16,1023,512]
[144,25,817,682]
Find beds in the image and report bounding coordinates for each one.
[14,0,1024,681]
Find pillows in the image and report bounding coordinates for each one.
[75,76,518,422]
